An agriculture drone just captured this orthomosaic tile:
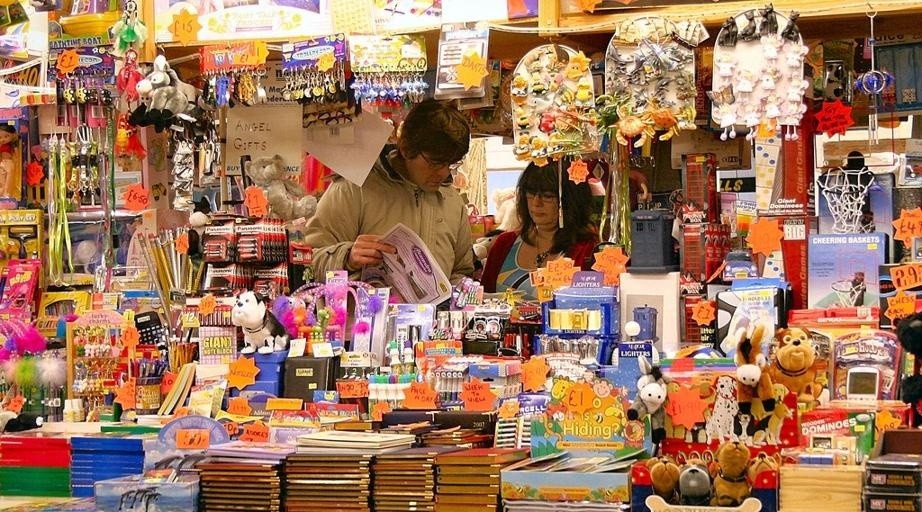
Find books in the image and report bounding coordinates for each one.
[378,223,457,309]
[157,360,192,418]
[1,413,647,511]
[162,363,197,416]
[172,360,202,416]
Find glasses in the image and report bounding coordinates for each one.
[419,149,462,171]
[523,190,555,203]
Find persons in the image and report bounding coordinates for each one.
[296,98,474,312]
[0,123,22,202]
[480,153,612,298]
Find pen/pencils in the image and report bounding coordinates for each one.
[129,223,205,385]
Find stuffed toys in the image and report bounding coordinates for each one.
[625,351,669,444]
[670,454,716,507]
[472,228,507,272]
[742,449,783,512]
[728,322,776,414]
[703,439,755,507]
[762,325,823,407]
[121,52,216,134]
[645,452,680,508]
[232,291,291,354]
[243,154,317,220]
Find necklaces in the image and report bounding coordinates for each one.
[531,234,554,268]
